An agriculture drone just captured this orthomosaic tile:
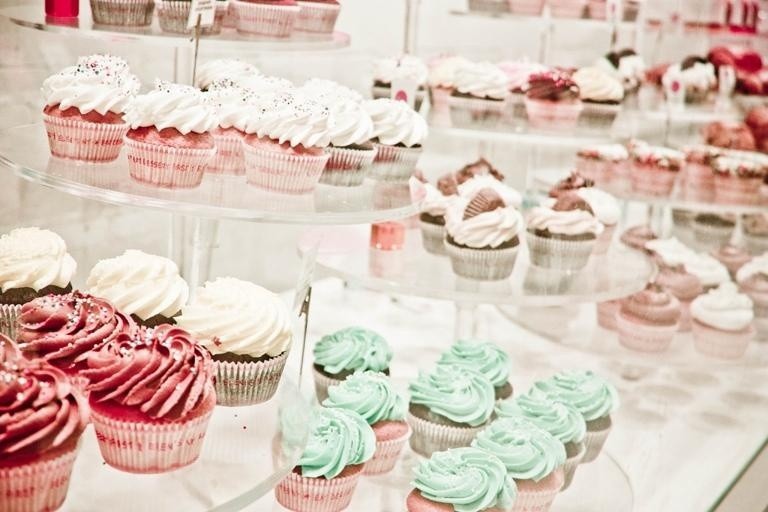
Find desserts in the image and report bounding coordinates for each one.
[273,330,622,512]
[0,0,768,355]
[0,228,296,512]
